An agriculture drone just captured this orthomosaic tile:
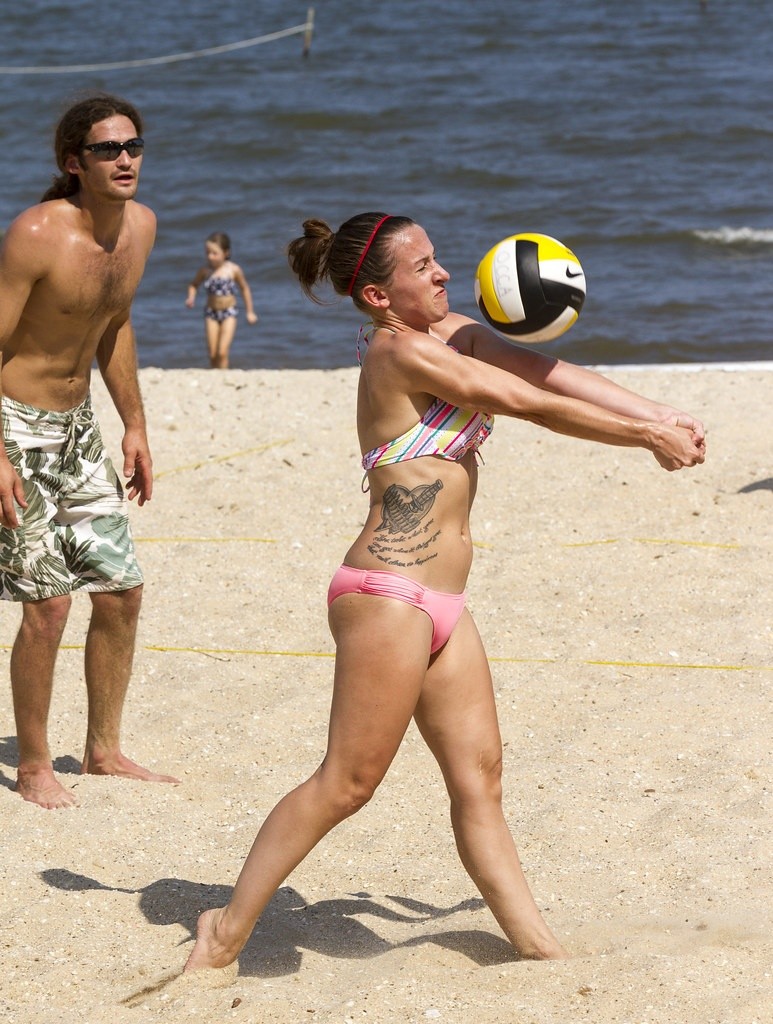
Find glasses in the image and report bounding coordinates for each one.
[81,137,145,161]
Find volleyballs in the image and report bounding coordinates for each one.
[474,230,587,345]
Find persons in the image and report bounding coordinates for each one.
[0,97,181,810]
[183,232,256,370]
[184,212,707,971]
